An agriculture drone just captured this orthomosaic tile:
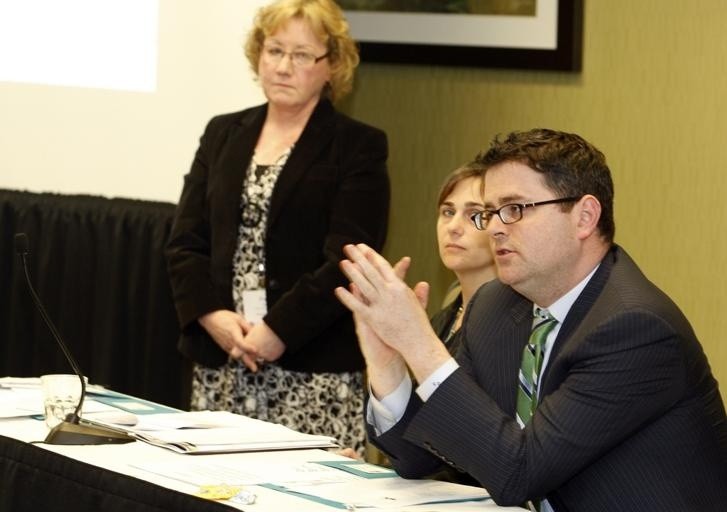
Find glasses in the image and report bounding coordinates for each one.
[265,46,330,70]
[471,197,578,230]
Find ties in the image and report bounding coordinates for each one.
[516,309,559,430]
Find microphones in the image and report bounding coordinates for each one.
[14,231,137,445]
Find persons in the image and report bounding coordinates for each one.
[164,0,392,459]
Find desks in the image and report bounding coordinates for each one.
[0,368,520,512]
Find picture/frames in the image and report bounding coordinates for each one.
[336,0,585,72]
